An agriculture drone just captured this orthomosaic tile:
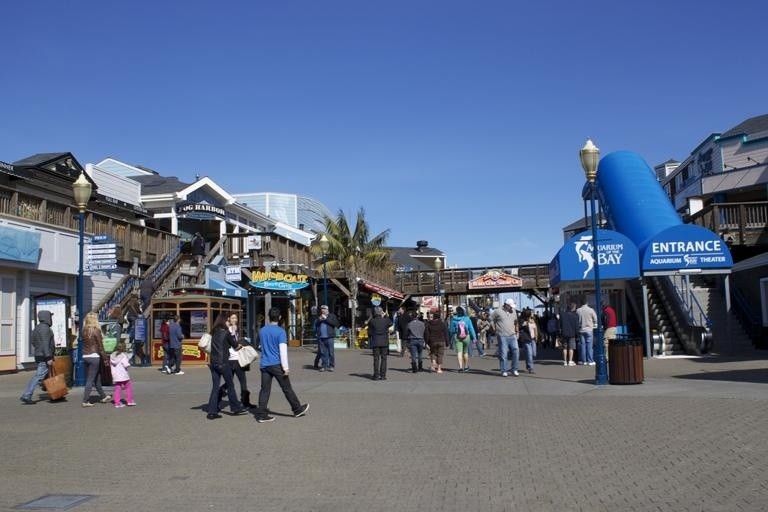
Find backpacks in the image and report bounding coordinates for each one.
[456,320,468,340]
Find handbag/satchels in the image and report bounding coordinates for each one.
[41,362,70,402]
[235,344,260,368]
[197,333,213,355]
[517,321,531,345]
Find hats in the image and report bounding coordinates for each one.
[319,305,329,310]
[504,298,518,309]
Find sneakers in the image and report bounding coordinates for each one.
[81,401,95,407]
[563,360,596,366]
[294,403,310,418]
[257,415,275,423]
[206,413,222,420]
[114,403,125,408]
[314,364,335,372]
[175,370,185,375]
[99,395,112,403]
[458,368,464,373]
[528,371,536,376]
[235,410,248,415]
[430,365,443,374]
[464,366,469,371]
[502,371,508,377]
[127,402,137,406]
[512,370,520,376]
[164,365,172,374]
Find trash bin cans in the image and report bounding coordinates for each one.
[100,353,113,385]
[608,339,643,384]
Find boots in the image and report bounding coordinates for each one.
[240,390,257,409]
[217,386,227,406]
[411,362,418,373]
[418,361,423,372]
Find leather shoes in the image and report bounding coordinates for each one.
[372,376,387,380]
[20,397,36,405]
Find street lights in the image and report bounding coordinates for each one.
[579,139,613,387]
[433,257,443,318]
[70,170,94,387]
[319,235,330,306]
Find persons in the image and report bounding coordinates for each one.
[159,317,173,373]
[312,316,325,368]
[364,300,558,358]
[81,311,113,407]
[256,307,311,423]
[574,297,598,366]
[19,310,67,405]
[206,313,248,418]
[192,236,206,267]
[367,306,392,381]
[108,340,137,408]
[448,307,478,372]
[190,231,203,265]
[212,311,258,412]
[557,301,580,367]
[404,312,426,373]
[508,308,537,374]
[164,315,186,375]
[489,298,521,377]
[110,274,157,343]
[423,313,446,374]
[314,305,340,372]
[600,300,617,364]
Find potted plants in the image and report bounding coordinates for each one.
[45,343,73,388]
